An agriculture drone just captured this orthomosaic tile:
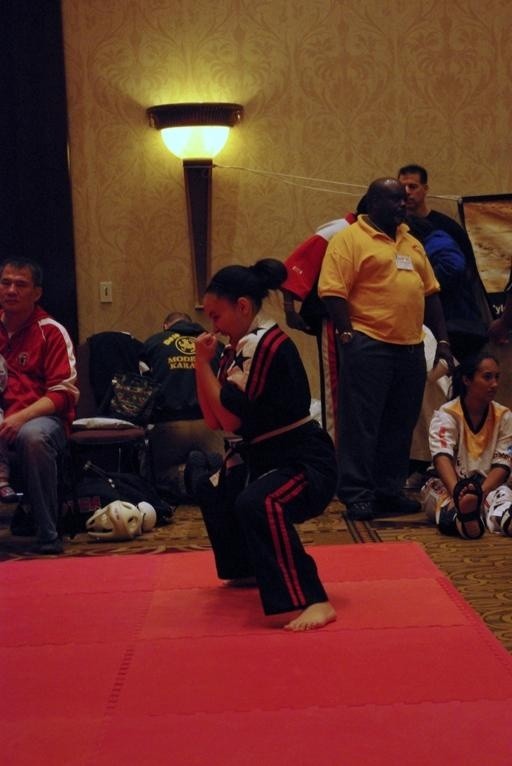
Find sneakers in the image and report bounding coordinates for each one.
[35,535,64,554]
[345,497,377,520]
[379,491,422,514]
[0,486,16,503]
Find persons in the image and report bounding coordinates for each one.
[417,351,512,538]
[140,313,227,510]
[317,177,456,519]
[398,164,489,459]
[194,259,339,632]
[0,258,80,552]
[279,193,369,451]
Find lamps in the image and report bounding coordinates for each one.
[144,102,245,311]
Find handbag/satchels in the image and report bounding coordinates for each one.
[97,371,162,426]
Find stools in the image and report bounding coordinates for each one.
[66,426,146,540]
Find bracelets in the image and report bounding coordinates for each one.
[341,332,352,337]
[439,340,450,346]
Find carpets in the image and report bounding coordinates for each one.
[1,537,512,766]
